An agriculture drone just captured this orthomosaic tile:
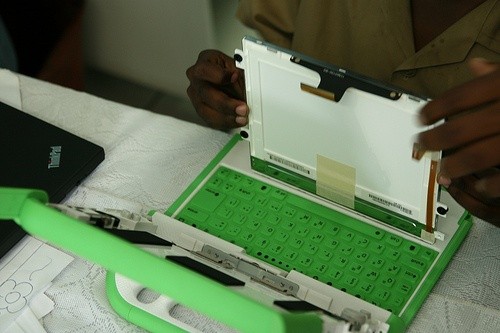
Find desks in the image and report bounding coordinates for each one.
[0,67,500,332]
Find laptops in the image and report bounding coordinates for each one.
[0,101,105,259]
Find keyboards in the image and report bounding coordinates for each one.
[165,133,474,333]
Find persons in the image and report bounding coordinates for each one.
[184,1,499,224]
